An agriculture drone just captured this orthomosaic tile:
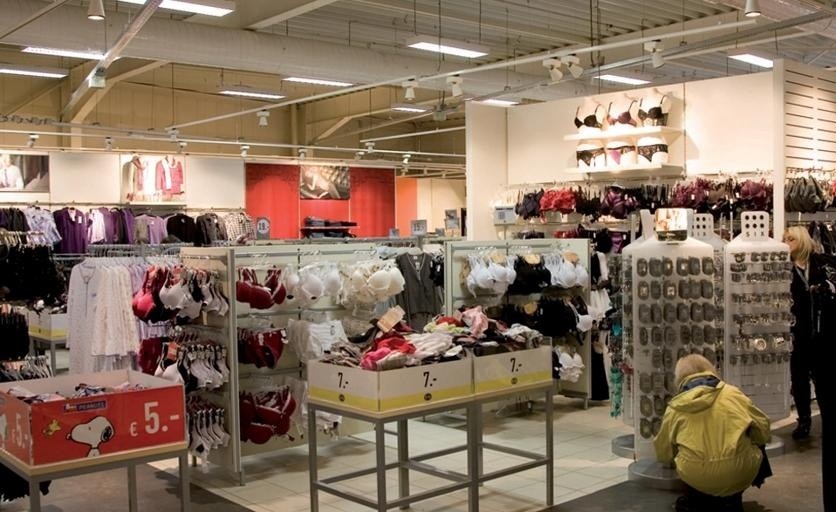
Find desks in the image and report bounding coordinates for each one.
[0,443,191,512]
[307,385,552,512]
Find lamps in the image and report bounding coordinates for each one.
[0,0,775,162]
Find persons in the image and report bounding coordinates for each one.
[636,86,673,168]
[153,154,185,202]
[604,91,642,169]
[574,92,608,170]
[652,354,773,511]
[125,154,151,203]
[783,224,836,440]
[299,167,340,200]
[0,153,25,191]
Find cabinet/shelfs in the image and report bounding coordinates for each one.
[556,126,684,181]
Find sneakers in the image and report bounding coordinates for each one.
[792,418,811,440]
[676,496,690,512]
